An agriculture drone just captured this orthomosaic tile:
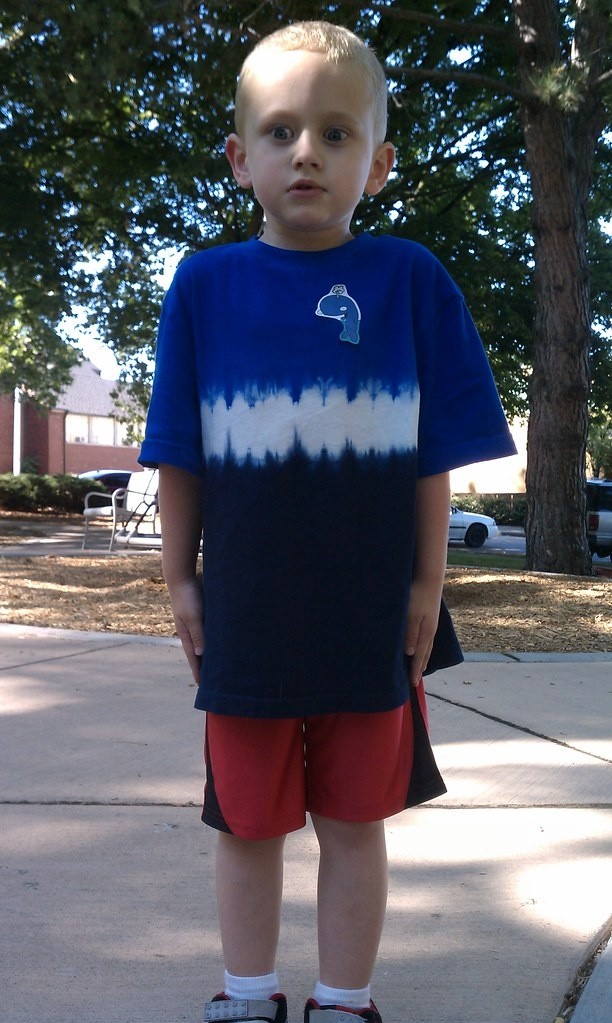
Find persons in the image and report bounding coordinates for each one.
[139,16,522,1022]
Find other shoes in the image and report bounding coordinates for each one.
[204,992,288,1023]
[303,998,383,1023]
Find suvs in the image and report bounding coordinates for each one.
[584,478,612,563]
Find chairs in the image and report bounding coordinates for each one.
[81,469,159,553]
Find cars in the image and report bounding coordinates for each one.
[447,503,501,548]
[78,470,135,508]
